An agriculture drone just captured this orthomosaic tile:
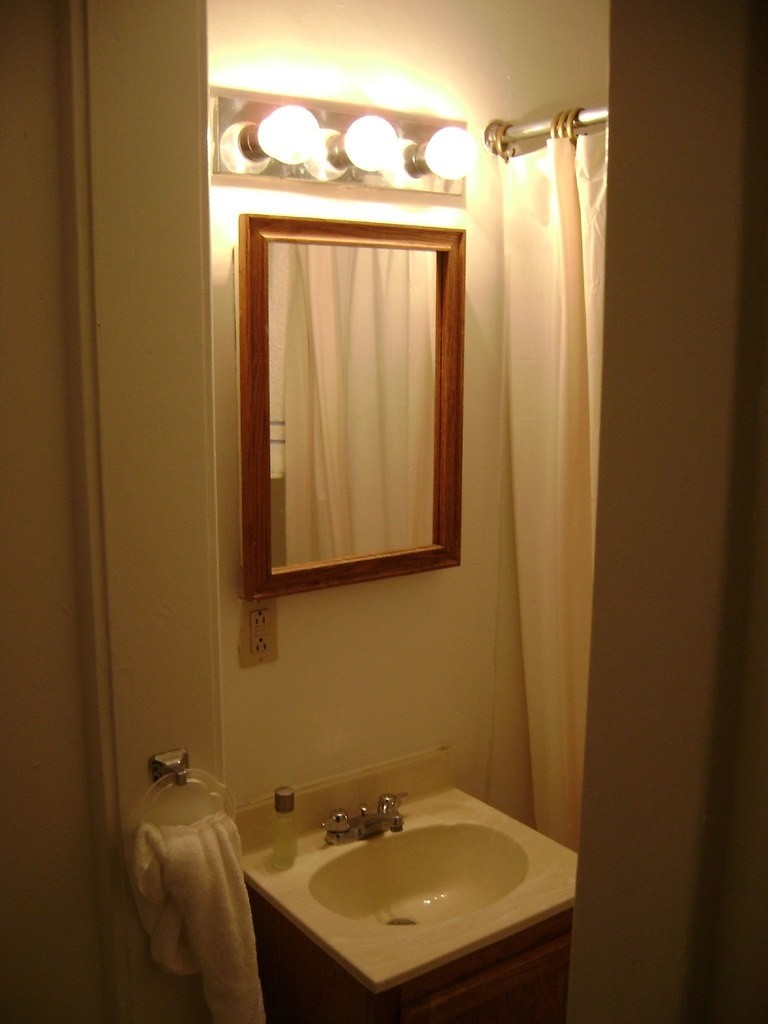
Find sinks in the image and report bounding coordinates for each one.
[305,821,532,926]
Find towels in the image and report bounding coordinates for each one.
[131,808,267,1024]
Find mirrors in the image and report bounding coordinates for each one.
[238,212,465,597]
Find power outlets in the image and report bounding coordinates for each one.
[241,599,278,667]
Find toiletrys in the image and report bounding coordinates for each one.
[269,785,298,871]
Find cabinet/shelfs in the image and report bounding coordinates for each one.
[246,888,573,1024]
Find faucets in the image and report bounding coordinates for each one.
[349,813,404,839]
